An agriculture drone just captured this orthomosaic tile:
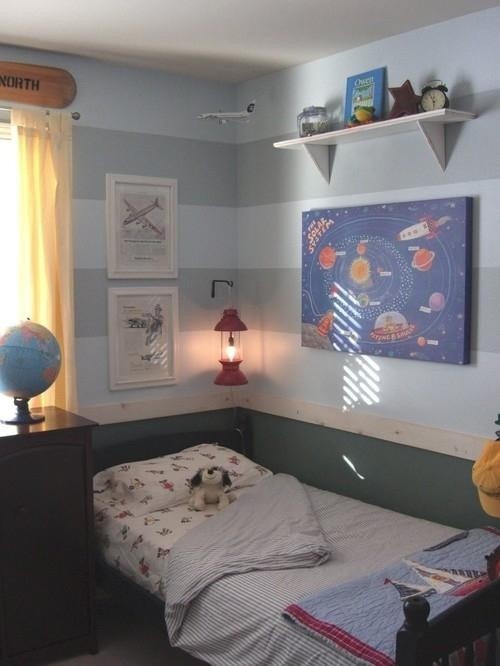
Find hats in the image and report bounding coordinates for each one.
[469,439,500,518]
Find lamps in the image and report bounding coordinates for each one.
[211,279,250,386]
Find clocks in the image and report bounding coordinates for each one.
[419,79,450,112]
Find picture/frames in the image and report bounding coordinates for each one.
[104,172,182,392]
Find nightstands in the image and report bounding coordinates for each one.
[2,407,100,666]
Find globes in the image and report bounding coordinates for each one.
[0,317,62,425]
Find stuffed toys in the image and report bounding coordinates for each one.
[189,464,233,513]
[348,104,376,126]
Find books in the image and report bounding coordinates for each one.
[342,66,387,129]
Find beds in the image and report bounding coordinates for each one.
[93,475,499,666]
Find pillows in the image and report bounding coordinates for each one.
[96,444,275,517]
